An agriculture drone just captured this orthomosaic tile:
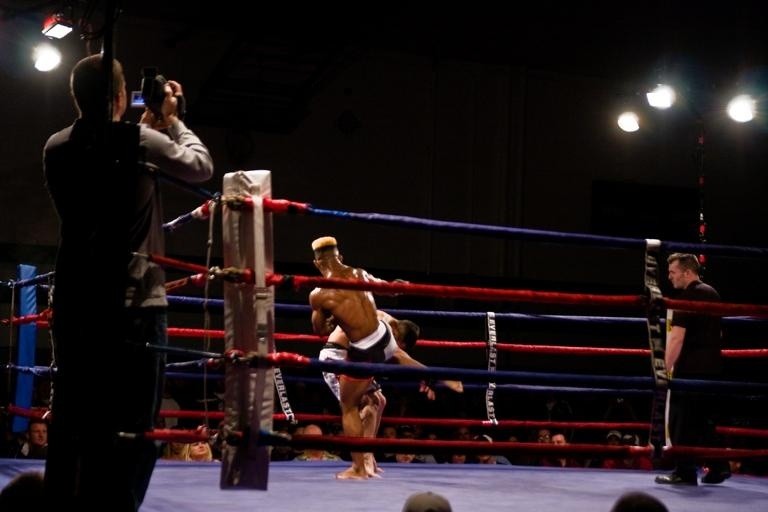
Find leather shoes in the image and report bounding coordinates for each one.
[702,471,731,483]
[655,471,697,485]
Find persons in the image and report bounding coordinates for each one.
[269,409,743,472]
[161,425,192,461]
[606,492,668,512]
[317,311,419,478]
[308,235,464,479]
[39,53,214,511]
[402,491,450,512]
[12,418,46,460]
[655,253,734,486]
[1,469,45,507]
[183,435,213,460]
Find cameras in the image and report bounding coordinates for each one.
[130,66,185,122]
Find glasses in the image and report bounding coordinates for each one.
[538,434,550,440]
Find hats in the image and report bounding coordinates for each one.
[621,434,640,446]
[606,430,621,440]
[472,434,492,442]
